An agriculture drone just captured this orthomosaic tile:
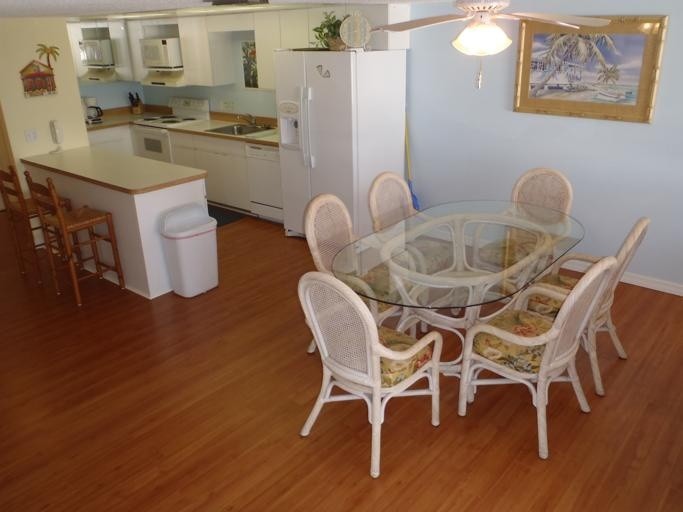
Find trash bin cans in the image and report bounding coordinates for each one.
[158,203,218,297]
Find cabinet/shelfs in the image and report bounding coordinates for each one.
[254,10,307,92]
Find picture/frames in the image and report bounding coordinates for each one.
[513,16,668,123]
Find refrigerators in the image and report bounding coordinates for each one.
[275,48,408,241]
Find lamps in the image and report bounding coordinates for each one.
[451,11,513,88]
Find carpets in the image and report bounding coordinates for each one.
[207,204,246,227]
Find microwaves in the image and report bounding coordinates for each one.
[77,40,114,69]
[138,39,183,72]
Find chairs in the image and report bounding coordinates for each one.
[368,173,419,233]
[24,170,126,306]
[457,256,618,459]
[511,169,574,216]
[0,166,83,284]
[520,216,650,396]
[297,272,442,478]
[304,192,354,354]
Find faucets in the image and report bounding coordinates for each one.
[235,113,257,125]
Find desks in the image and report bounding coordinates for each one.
[331,199,586,404]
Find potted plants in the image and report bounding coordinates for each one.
[309,10,347,50]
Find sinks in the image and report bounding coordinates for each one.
[203,124,265,136]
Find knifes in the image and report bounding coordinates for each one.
[127,92,141,107]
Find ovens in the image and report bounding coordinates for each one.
[129,126,171,162]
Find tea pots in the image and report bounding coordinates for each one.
[87,106,103,121]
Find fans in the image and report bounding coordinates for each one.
[374,0,612,34]
